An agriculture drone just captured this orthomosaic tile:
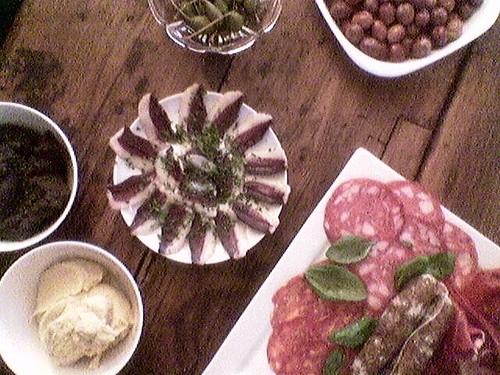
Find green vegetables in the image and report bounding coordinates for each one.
[303,235,455,375]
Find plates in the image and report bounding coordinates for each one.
[147,0,283,55]
[200,147,500,374]
[314,0,500,78]
[111,91,288,261]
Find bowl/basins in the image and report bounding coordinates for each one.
[0,102,78,251]
[0,241,144,375]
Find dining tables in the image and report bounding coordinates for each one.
[0,1,500,375]
